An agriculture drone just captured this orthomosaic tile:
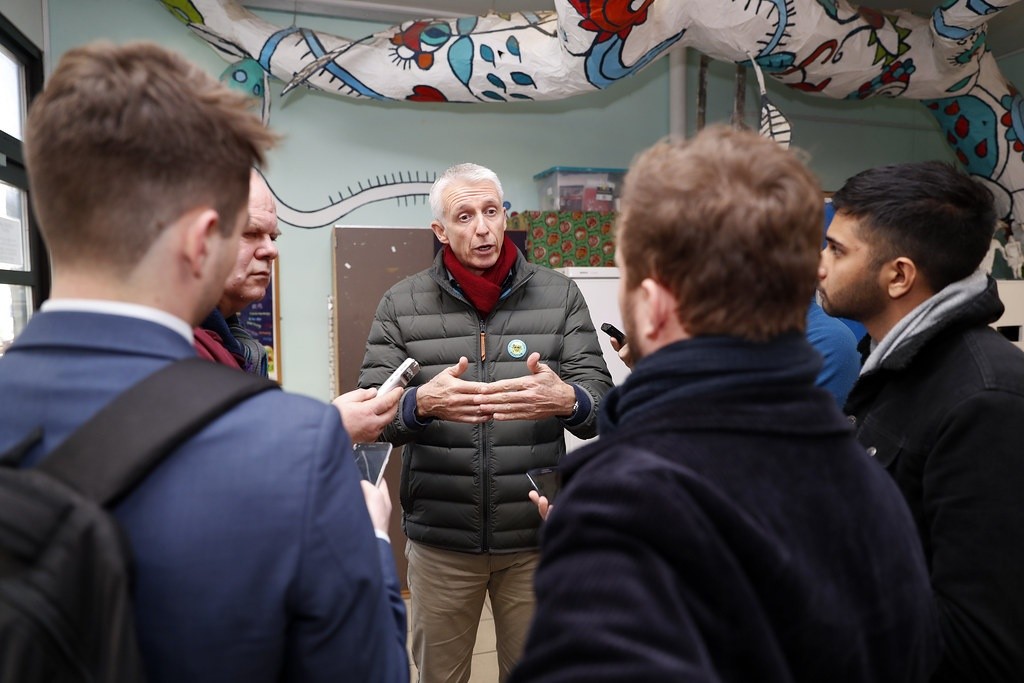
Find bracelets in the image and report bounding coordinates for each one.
[572,401,578,415]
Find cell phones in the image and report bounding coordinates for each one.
[354,442,393,488]
[527,465,562,506]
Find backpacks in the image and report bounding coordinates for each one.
[0,356,280,683]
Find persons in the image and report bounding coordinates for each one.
[0,36,410,683]
[187,162,402,447]
[357,163,615,682]
[815,161,1024,683]
[609,301,861,416]
[511,125,932,683]
